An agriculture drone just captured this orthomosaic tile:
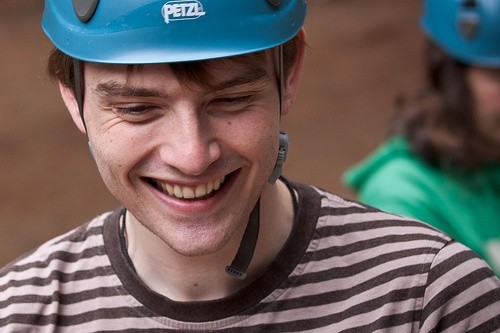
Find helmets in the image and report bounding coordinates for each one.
[420,0,500,69]
[41,0,308,64]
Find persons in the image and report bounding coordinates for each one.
[342,0,500,280]
[0,0,500,333]
